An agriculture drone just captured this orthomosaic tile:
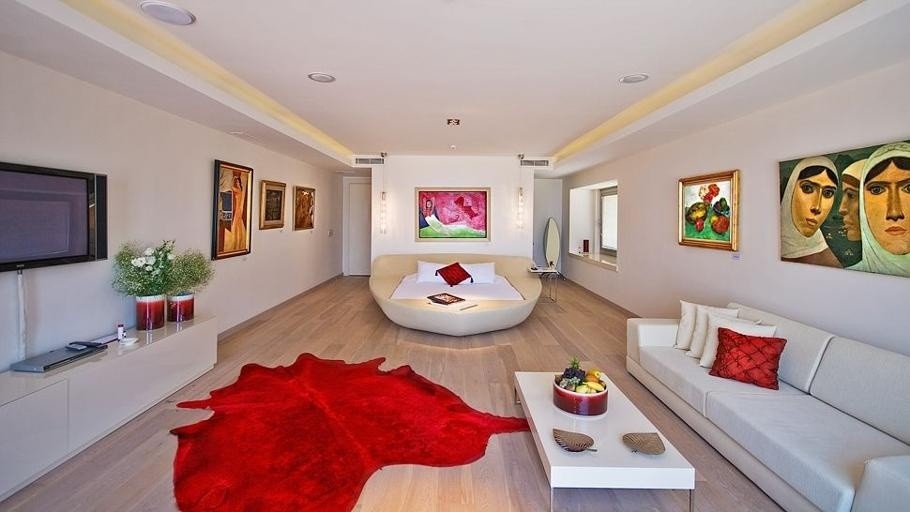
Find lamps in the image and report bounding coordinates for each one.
[518,154,525,196]
[380,152,387,200]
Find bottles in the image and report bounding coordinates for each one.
[117,323,124,343]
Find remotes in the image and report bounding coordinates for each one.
[65,344,87,350]
[69,341,102,347]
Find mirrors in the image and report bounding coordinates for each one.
[540,216,567,282]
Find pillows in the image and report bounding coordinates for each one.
[672,299,787,391]
[415,260,496,288]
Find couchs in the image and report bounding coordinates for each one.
[626,301,910,512]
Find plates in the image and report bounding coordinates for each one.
[713,204,731,215]
[553,428,597,453]
[120,338,139,345]
[623,432,666,456]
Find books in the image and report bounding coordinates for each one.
[425,292,479,312]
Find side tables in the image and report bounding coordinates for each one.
[528,267,558,304]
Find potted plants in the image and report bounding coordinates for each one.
[164,248,216,323]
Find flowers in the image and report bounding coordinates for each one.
[110,237,179,301]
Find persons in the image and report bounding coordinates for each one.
[231,177,246,249]
[781,141,910,278]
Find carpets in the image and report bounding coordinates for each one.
[168,352,531,512]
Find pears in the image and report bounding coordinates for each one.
[587,367,601,380]
[576,385,590,394]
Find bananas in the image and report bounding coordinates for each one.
[582,382,604,392]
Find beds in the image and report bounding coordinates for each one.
[368,253,543,338]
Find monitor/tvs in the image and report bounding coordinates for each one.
[0,163,108,272]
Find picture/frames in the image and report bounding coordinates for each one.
[259,179,286,230]
[414,186,491,242]
[211,159,254,260]
[292,185,317,231]
[676,169,740,251]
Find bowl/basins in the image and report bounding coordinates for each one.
[553,376,608,416]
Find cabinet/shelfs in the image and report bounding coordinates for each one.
[70,314,216,466]
[0,368,69,504]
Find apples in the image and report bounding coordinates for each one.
[585,374,599,383]
[713,215,729,233]
[566,385,576,391]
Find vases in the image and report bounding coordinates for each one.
[135,293,167,330]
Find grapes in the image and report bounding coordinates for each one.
[560,358,585,388]
